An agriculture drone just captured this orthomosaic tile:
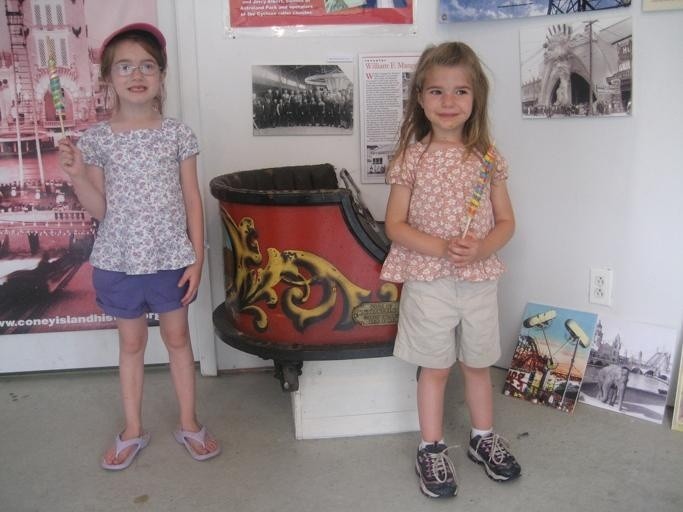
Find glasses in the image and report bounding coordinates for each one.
[110,63,162,77]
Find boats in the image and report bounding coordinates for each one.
[0,130,58,157]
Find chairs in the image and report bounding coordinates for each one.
[212,165,420,440]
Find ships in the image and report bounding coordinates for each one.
[582,351,671,398]
[0,180,100,253]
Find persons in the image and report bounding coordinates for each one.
[526,99,632,119]
[57,21,222,472]
[0,99,71,221]
[379,40,522,500]
[254,80,353,129]
[616,366,629,411]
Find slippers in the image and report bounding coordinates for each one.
[102,430,151,469]
[177,424,220,461]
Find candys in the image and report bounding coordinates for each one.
[461,140,496,242]
[48,55,67,138]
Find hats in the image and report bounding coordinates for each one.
[99,23,167,58]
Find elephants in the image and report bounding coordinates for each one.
[597,363,629,411]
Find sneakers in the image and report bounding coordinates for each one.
[469,430,521,482]
[417,443,457,498]
